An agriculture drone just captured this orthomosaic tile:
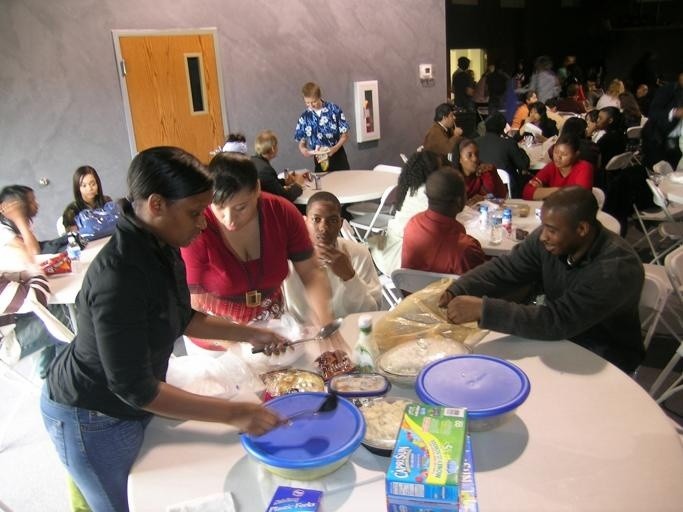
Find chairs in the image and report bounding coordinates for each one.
[648,339,682,441]
[627,125,642,138]
[391,267,461,300]
[352,183,401,244]
[591,184,608,209]
[596,210,622,236]
[345,164,400,215]
[662,246,683,303]
[615,177,682,257]
[623,269,667,376]
[606,149,633,171]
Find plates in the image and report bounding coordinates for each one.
[256,368,327,400]
[308,148,331,155]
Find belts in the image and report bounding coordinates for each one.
[228,289,274,308]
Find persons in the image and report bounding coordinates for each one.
[438,186,644,375]
[224,134,246,155]
[281,191,381,322]
[1,185,39,274]
[400,169,489,305]
[371,151,452,276]
[63,166,112,249]
[0,271,75,380]
[422,51,683,200]
[294,81,350,172]
[250,130,304,203]
[41,145,296,512]
[180,152,354,369]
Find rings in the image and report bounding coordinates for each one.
[327,260,333,264]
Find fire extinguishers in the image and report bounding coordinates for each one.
[365,98,372,132]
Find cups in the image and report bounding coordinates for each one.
[284,167,289,178]
[524,135,533,148]
[289,168,295,176]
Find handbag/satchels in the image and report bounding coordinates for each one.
[75,202,117,240]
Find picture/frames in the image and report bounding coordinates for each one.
[353,80,380,144]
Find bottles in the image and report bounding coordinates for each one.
[353,315,380,374]
[67,236,81,272]
[478,206,512,244]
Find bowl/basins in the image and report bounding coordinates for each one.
[415,353,532,433]
[377,335,474,386]
[238,391,367,482]
[359,396,417,456]
